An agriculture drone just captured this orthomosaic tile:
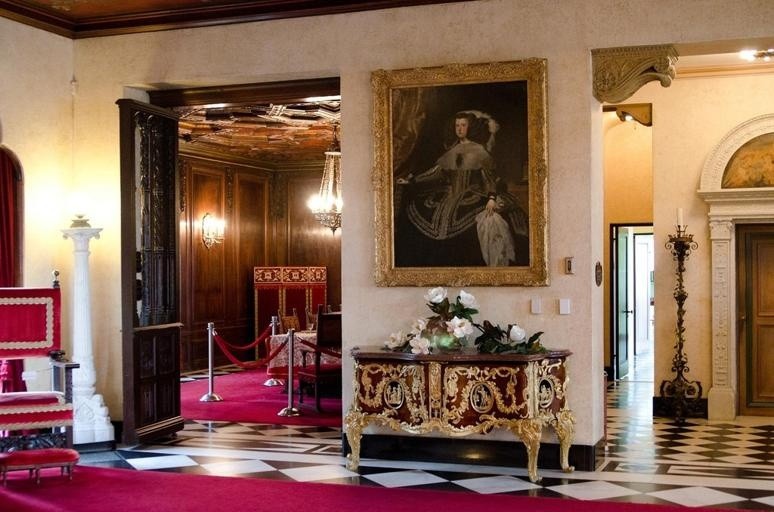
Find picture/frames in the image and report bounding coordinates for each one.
[363,52,551,288]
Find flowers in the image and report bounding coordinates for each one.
[375,281,555,357]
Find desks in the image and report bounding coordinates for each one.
[264,329,340,396]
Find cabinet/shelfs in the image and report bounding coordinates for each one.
[340,342,577,483]
[250,262,326,362]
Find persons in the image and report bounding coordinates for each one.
[393,108,529,267]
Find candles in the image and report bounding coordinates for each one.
[673,206,686,240]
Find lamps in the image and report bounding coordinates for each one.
[58,184,116,449]
[736,44,773,67]
[303,120,341,238]
[201,212,228,251]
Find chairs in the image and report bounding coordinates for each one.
[0,265,81,489]
[296,302,342,414]
[273,302,341,333]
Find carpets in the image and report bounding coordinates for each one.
[177,363,343,430]
[0,465,774,512]
